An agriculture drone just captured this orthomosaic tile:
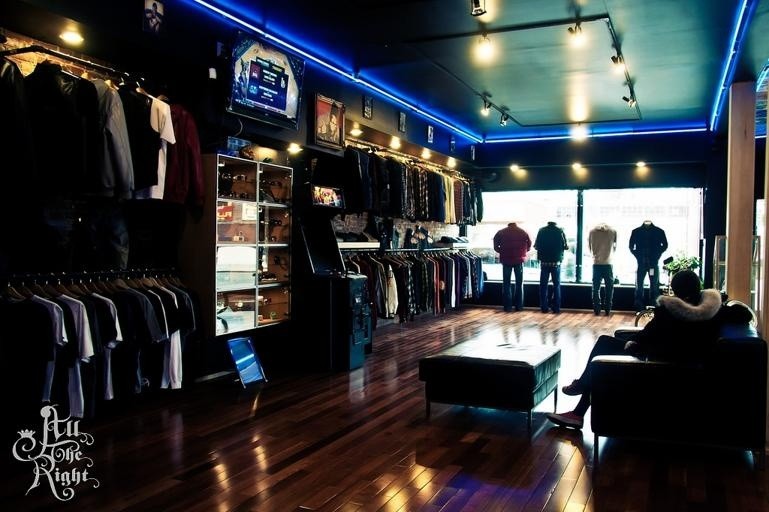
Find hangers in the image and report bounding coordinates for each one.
[342,247,471,261]
[0,267,177,298]
[343,135,466,178]
[0,36,171,100]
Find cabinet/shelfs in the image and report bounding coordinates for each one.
[713,235,760,321]
[175,153,293,336]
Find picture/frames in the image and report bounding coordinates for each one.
[312,91,345,151]
[470,145,476,161]
[472,0,486,17]
[312,183,346,210]
[450,135,455,153]
[143,0,164,32]
[398,111,406,133]
[363,94,373,120]
[427,126,434,143]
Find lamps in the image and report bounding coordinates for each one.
[622,96,636,108]
[611,56,623,71]
[481,102,492,116]
[567,24,584,41]
[499,115,509,127]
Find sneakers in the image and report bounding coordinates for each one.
[562,380,583,395]
[547,412,583,428]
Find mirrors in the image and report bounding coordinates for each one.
[226,337,268,389]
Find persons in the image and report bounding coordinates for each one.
[493,222,531,313]
[588,224,617,316]
[533,221,568,313]
[545,270,753,428]
[629,220,668,313]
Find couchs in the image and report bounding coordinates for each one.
[591,321,769,470]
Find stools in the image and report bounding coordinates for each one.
[420,338,561,437]
